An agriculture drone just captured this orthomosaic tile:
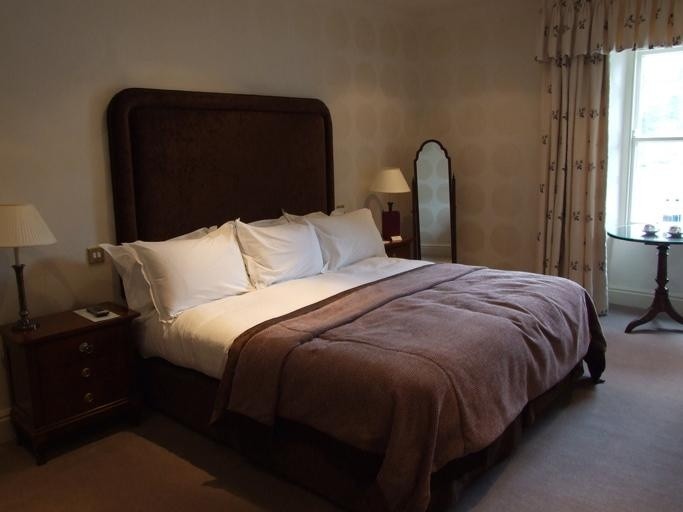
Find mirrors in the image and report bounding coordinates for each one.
[412,139,457,264]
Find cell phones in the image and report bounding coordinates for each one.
[87,306,109,317]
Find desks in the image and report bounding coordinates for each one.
[607,223,683,333]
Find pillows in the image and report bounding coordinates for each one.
[231,216,325,287]
[101,225,218,322]
[310,207,387,274]
[234,212,288,282]
[121,220,255,321]
[281,202,347,266]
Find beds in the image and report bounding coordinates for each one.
[107,86,609,510]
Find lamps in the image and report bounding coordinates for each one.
[0,203,57,332]
[369,168,412,239]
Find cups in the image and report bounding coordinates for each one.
[645,224,655,232]
[670,225,681,234]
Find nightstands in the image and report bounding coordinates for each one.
[382,236,412,258]
[2,301,141,467]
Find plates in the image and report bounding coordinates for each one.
[667,231,683,238]
[642,230,658,234]
